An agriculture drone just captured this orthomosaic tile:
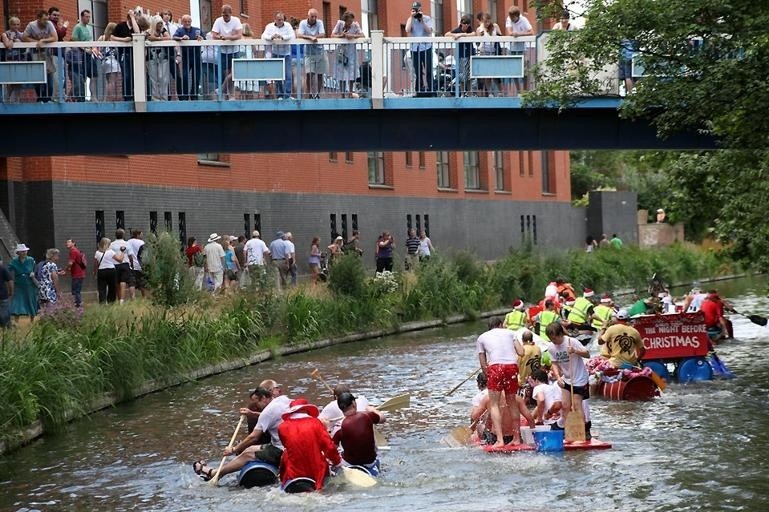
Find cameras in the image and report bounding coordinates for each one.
[414,14,422,19]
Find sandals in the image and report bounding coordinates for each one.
[194,458,213,484]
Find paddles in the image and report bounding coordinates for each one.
[710,345,736,379]
[341,464,377,489]
[564,354,585,443]
[324,393,410,424]
[723,307,767,326]
[312,368,388,447]
[637,359,666,391]
[450,408,487,445]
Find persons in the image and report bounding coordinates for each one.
[620,40,634,98]
[472,278,736,453]
[95,229,299,304]
[0,6,370,99]
[403,2,534,97]
[599,233,608,249]
[192,377,388,489]
[374,228,434,277]
[310,230,364,282]
[554,12,575,29]
[6,238,87,321]
[585,236,597,253]
[609,233,622,249]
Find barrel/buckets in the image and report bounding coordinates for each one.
[589,383,601,399]
[520,425,551,444]
[283,478,319,492]
[674,357,712,384]
[642,359,670,385]
[603,375,658,401]
[532,431,564,451]
[238,460,280,489]
[347,460,380,479]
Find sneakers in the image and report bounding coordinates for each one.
[211,88,324,99]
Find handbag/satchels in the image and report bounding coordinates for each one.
[80,251,88,269]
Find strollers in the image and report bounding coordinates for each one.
[318,250,330,281]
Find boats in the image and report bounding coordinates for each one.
[231,450,382,498]
[470,316,736,456]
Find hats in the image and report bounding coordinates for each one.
[230,236,238,241]
[337,392,358,410]
[599,295,612,303]
[208,233,221,243]
[15,243,30,254]
[583,288,595,299]
[512,300,524,310]
[281,398,319,420]
[616,310,630,320]
[275,231,285,238]
[252,230,260,236]
[337,236,343,240]
